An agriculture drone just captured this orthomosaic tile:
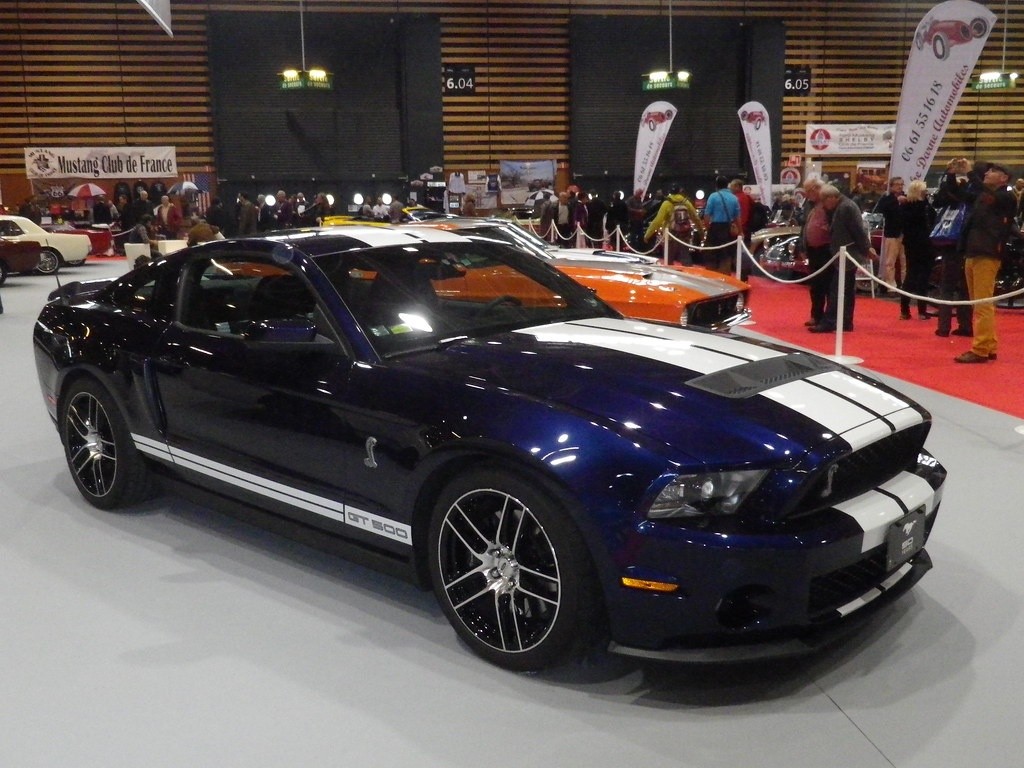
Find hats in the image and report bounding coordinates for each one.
[140,213,153,220]
[986,159,1013,180]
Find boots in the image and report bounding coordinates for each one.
[951,305,974,336]
[935,312,952,337]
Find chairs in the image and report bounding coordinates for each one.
[247,263,445,336]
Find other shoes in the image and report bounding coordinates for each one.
[807,320,833,333]
[832,322,854,331]
[953,350,988,363]
[803,316,815,326]
[899,314,911,319]
[919,313,931,319]
[987,352,997,360]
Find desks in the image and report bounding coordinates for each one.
[56,229,112,257]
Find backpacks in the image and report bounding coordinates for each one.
[663,194,693,234]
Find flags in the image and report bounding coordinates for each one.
[183,173,211,216]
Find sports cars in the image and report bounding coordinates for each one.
[32,225,949,672]
[208,215,756,335]
[318,201,444,232]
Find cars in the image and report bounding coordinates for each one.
[39,226,113,268]
[0,233,43,289]
[745,204,1024,313]
[0,214,92,277]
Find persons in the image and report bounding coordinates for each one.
[463,194,476,216]
[524,158,1024,363]
[20,190,419,256]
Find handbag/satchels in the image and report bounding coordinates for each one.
[745,195,772,231]
[729,222,739,236]
[929,203,966,246]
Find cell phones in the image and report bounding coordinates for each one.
[954,161,965,169]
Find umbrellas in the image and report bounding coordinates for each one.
[67,183,106,205]
[525,189,560,206]
[167,181,203,196]
